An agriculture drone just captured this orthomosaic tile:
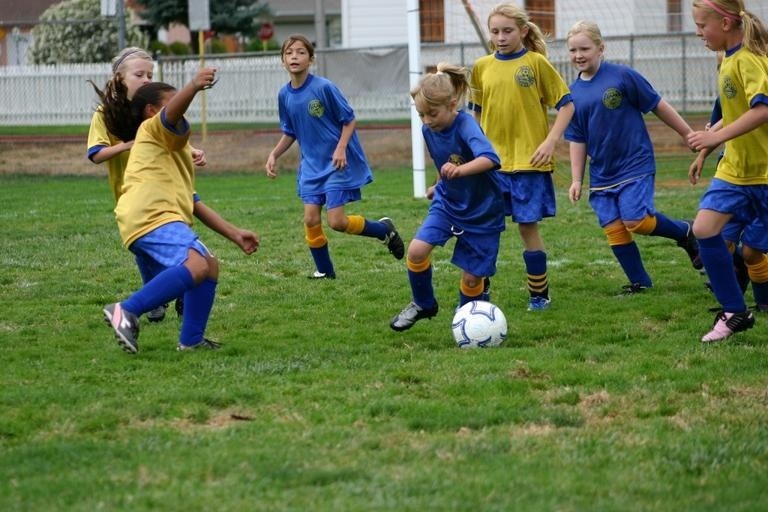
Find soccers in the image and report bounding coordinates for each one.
[452,300,507,348]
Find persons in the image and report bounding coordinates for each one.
[687,48,767,310]
[691,0,768,343]
[466,2,574,316]
[102,66,259,354]
[86,46,207,323]
[265,35,405,280]
[564,20,707,295]
[390,59,508,332]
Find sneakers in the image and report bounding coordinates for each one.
[101,301,225,355]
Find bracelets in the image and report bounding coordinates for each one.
[191,79,204,93]
